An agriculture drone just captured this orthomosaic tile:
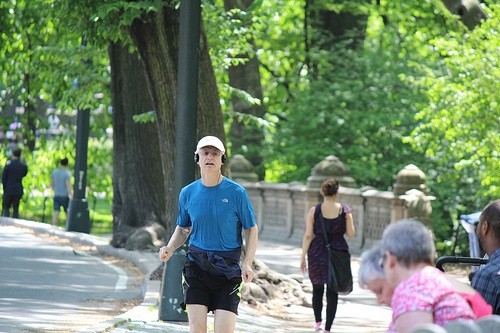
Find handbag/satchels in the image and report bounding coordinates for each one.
[328,249,353,295]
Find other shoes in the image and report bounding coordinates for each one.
[315,325,322,330]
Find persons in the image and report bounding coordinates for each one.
[159,135,258,333]
[1,147,28,219]
[357,198,500,333]
[50,157,73,228]
[300,178,357,333]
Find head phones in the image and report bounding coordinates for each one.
[194,153,227,164]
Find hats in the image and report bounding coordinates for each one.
[195,136,225,155]
